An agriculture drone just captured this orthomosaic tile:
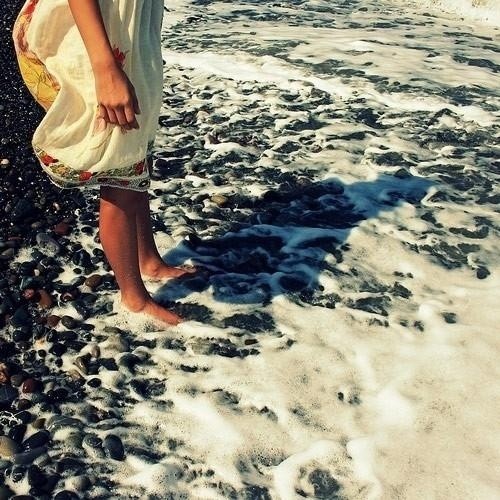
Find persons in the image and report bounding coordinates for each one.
[11,0,196,326]
